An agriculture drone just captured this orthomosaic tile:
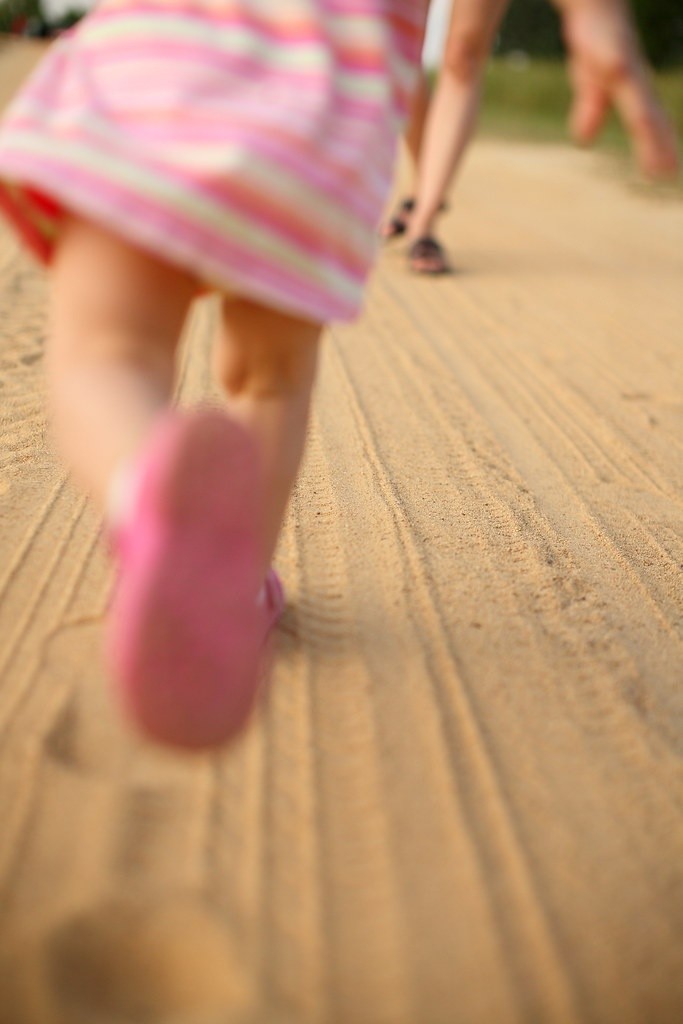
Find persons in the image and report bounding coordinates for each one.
[377,0,683,277]
[0,0,409,758]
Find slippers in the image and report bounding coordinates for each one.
[404,240,447,276]
[376,195,415,239]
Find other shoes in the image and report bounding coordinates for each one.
[258,568,283,644]
[104,399,264,747]
